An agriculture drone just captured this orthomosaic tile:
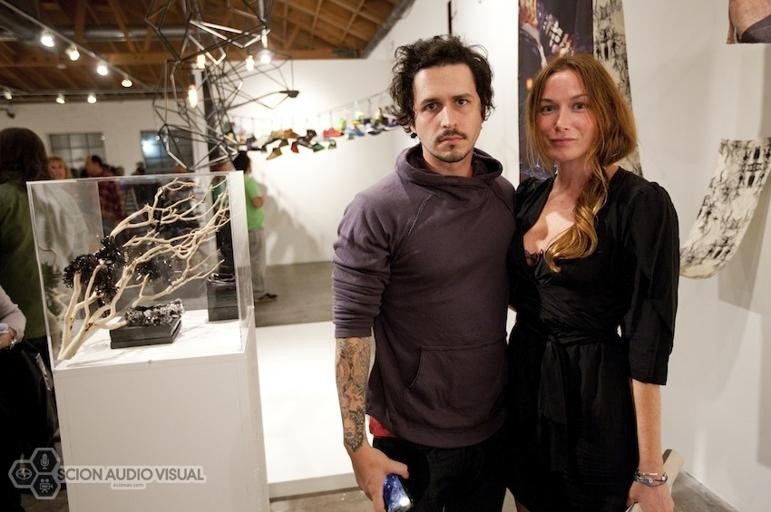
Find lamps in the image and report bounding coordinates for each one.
[2,28,132,104]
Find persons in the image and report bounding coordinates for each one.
[0,127,191,512]
[212,157,237,282]
[233,152,279,301]
[506,53,677,512]
[329,32,515,512]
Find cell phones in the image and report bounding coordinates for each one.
[383,473,416,512]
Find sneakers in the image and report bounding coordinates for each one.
[255,293,278,303]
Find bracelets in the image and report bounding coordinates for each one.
[633,471,669,488]
